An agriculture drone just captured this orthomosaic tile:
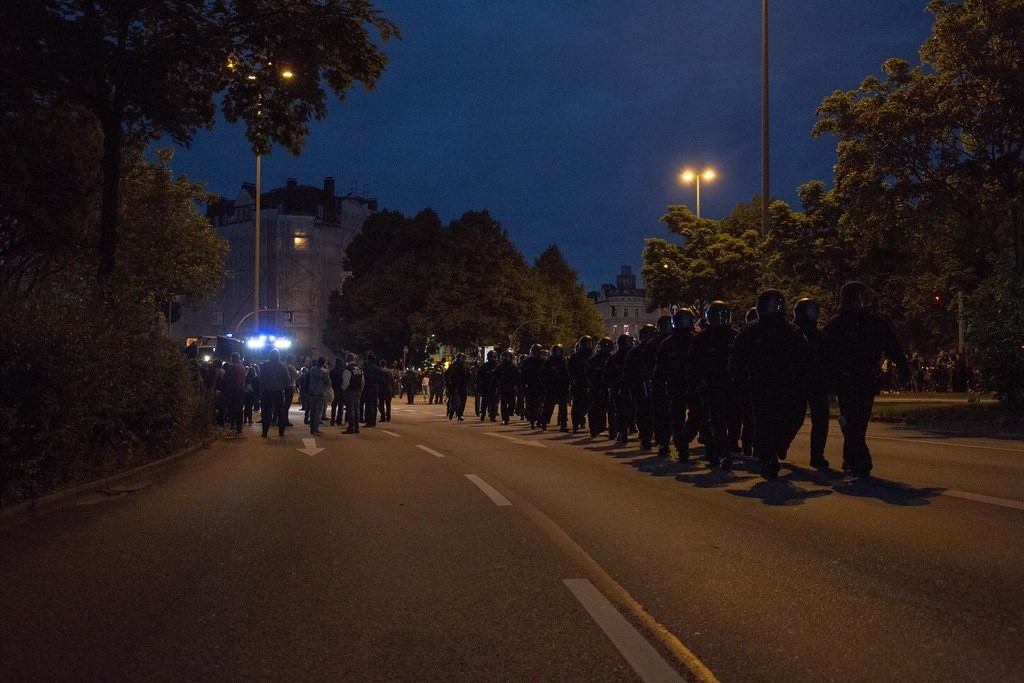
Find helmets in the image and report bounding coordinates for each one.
[446,280,874,369]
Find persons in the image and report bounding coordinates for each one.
[199,348,403,437]
[402,281,907,482]
[878,347,974,392]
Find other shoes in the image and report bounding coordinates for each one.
[261,432,267,437]
[342,431,352,433]
[355,430,359,433]
[447,404,870,483]
[314,431,324,436]
[279,432,284,436]
[362,424,371,427]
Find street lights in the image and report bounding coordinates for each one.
[227,57,294,334]
[683,165,716,220]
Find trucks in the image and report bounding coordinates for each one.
[185,333,246,384]
